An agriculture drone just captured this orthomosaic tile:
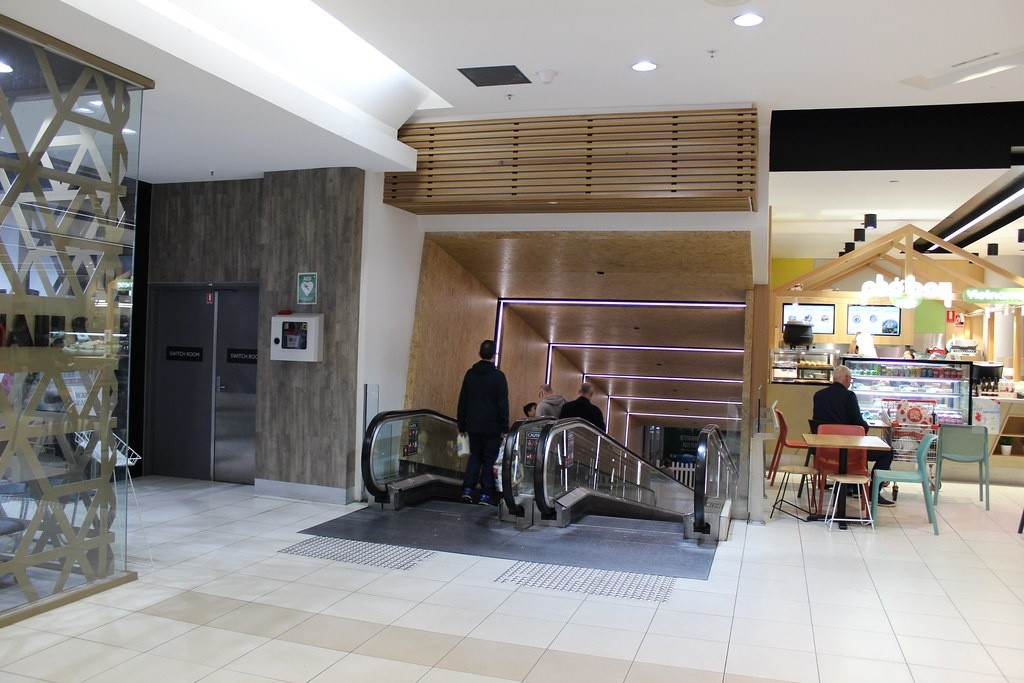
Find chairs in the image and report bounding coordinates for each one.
[811,425,870,514]
[797,420,832,498]
[871,434,940,536]
[766,409,808,486]
[934,424,990,510]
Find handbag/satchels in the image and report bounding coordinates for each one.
[893,400,933,439]
[492,438,524,492]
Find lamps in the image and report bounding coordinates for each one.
[987,243,998,256]
[838,214,876,256]
[792,289,797,305]
[969,253,979,264]
[1018,228,1024,243]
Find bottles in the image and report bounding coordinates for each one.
[971,377,978,397]
[981,376,1014,396]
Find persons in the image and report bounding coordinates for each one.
[903,351,915,360]
[810,365,896,508]
[558,382,606,486]
[523,402,537,417]
[523,384,566,485]
[848,332,862,354]
[456,340,510,508]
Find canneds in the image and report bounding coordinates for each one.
[874,363,963,379]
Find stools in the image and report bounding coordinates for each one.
[826,474,874,531]
[769,466,817,522]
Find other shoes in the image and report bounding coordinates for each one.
[478,492,497,507]
[462,488,472,503]
[869,494,896,506]
[852,488,862,497]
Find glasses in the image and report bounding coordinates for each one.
[846,376,853,382]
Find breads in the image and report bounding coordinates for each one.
[799,360,827,366]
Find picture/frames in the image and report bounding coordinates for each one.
[846,304,900,337]
[782,303,834,335]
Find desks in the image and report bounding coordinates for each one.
[801,434,891,530]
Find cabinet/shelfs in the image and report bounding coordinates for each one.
[770,349,831,385]
[841,357,973,426]
